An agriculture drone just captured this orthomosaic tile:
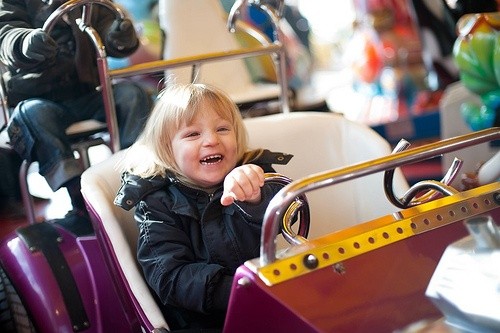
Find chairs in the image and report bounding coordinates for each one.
[0,62,110,226]
[158,0,297,113]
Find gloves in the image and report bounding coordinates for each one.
[20,29,58,62]
[108,18,138,49]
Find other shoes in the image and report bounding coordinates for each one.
[55,209,93,236]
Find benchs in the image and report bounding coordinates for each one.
[79,108,424,333]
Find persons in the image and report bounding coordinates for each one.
[115,83,297,333]
[0,0,152,237]
